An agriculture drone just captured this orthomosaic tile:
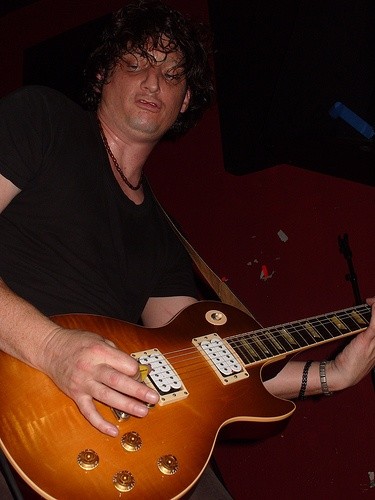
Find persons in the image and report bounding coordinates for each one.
[0,1,375,499]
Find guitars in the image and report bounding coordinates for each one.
[0,300,373,499]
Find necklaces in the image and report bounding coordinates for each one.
[98,125,145,192]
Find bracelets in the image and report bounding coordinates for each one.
[296,358,310,403]
[318,359,332,398]
